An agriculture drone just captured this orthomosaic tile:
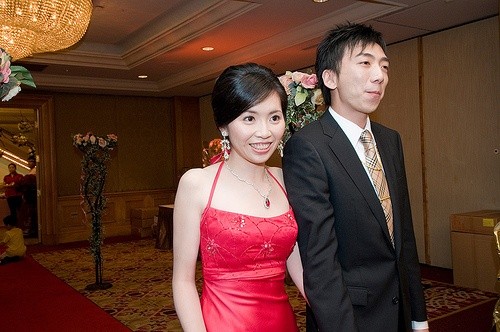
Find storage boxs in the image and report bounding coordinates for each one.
[448,208,500,293]
[133,206,159,237]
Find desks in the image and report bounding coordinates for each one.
[155,204,175,250]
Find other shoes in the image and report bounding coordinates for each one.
[23,232,37,239]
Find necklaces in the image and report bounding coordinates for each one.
[224,160,272,209]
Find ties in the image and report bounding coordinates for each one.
[358,130,395,251]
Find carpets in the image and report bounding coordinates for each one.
[29,240,497,332]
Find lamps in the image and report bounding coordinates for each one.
[0,0,94,61]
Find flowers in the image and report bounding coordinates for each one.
[71,131,117,264]
[277,70,326,157]
[202,138,225,166]
[0,50,36,101]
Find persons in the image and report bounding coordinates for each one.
[283,18,429,332]
[2,155,39,238]
[171,62,310,332]
[0,215,27,264]
